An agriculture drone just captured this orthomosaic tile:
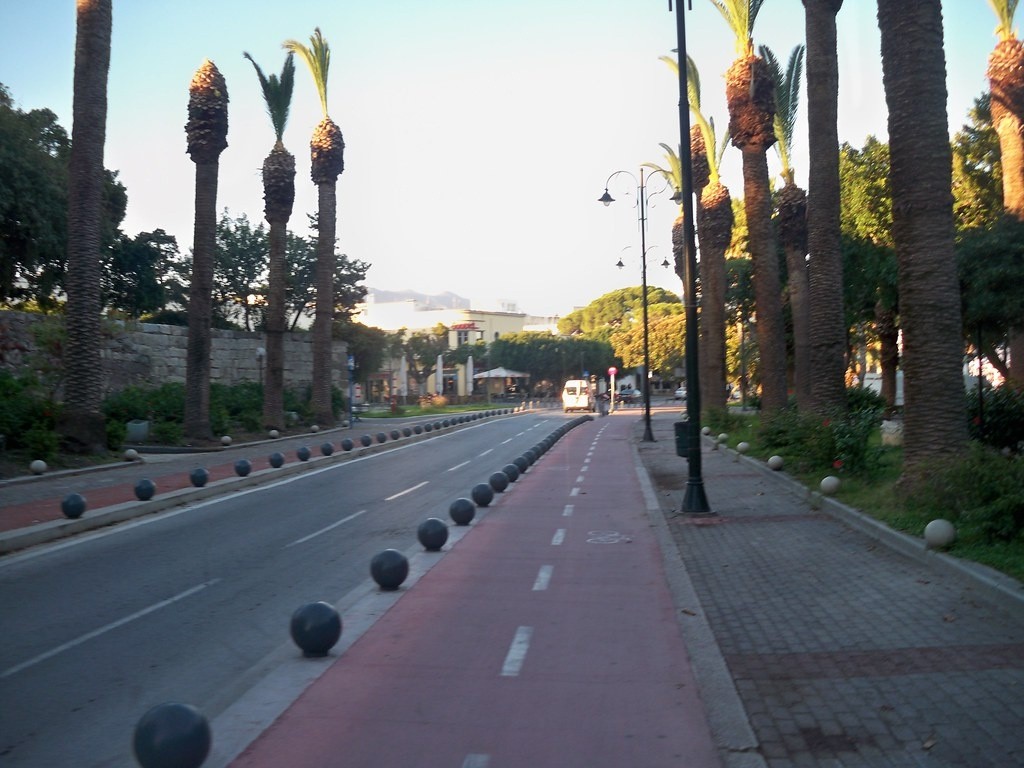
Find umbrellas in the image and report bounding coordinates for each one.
[471,366,530,394]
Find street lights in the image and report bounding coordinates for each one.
[598,167,686,443]
[617,246,672,440]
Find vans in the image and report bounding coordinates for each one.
[561,378,597,412]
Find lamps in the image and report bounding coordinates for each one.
[891,414,904,425]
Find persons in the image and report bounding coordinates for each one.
[607,382,632,395]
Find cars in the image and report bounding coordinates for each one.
[617,388,644,405]
[725,383,741,400]
[675,386,688,401]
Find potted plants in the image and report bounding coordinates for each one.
[123,394,152,440]
